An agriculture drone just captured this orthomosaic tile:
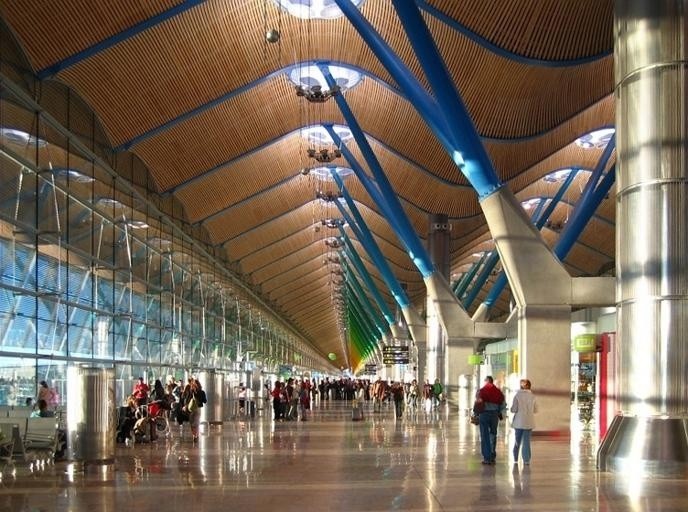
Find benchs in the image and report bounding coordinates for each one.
[1,405,66,479]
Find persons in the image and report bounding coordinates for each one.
[511,380,538,465]
[26,381,67,461]
[476,376,504,464]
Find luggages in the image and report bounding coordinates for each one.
[352,400,363,420]
[133,404,151,444]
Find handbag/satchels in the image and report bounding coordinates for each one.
[188,392,199,413]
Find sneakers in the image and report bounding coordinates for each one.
[514,458,530,465]
[193,435,199,442]
[482,459,496,465]
